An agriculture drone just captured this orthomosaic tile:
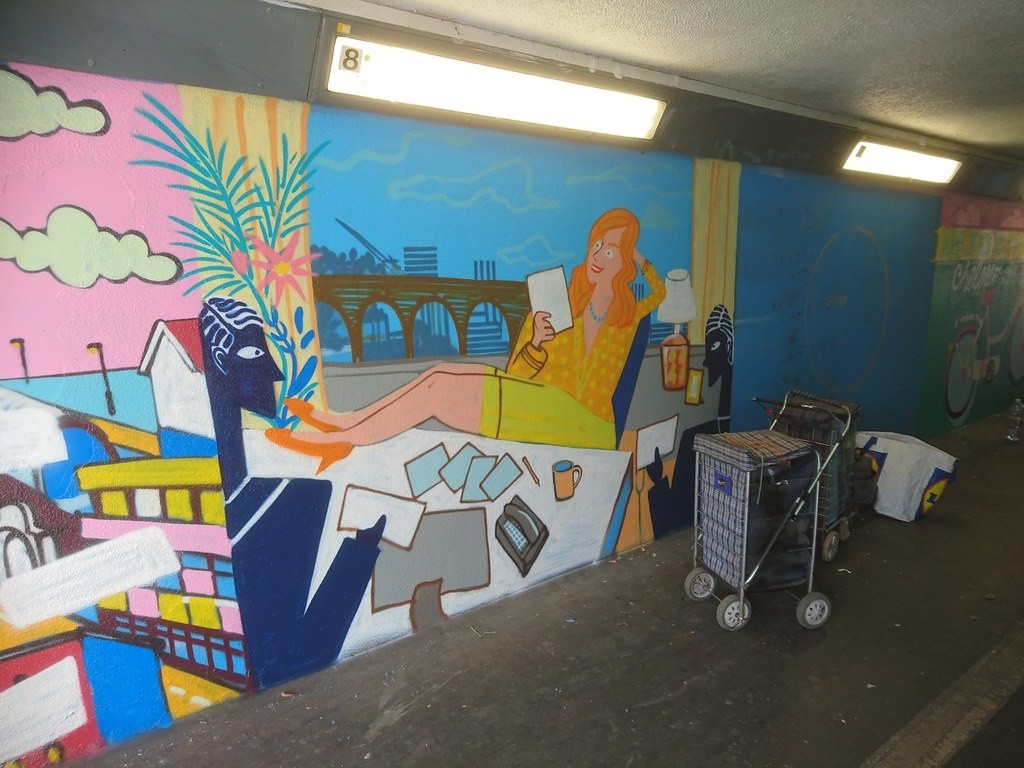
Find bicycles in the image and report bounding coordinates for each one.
[944,281,1024,429]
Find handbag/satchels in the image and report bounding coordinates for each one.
[854,431,959,522]
[854,459,877,504]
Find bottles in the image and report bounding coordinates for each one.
[1007,397,1024,443]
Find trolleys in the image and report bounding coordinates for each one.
[683,388,853,632]
